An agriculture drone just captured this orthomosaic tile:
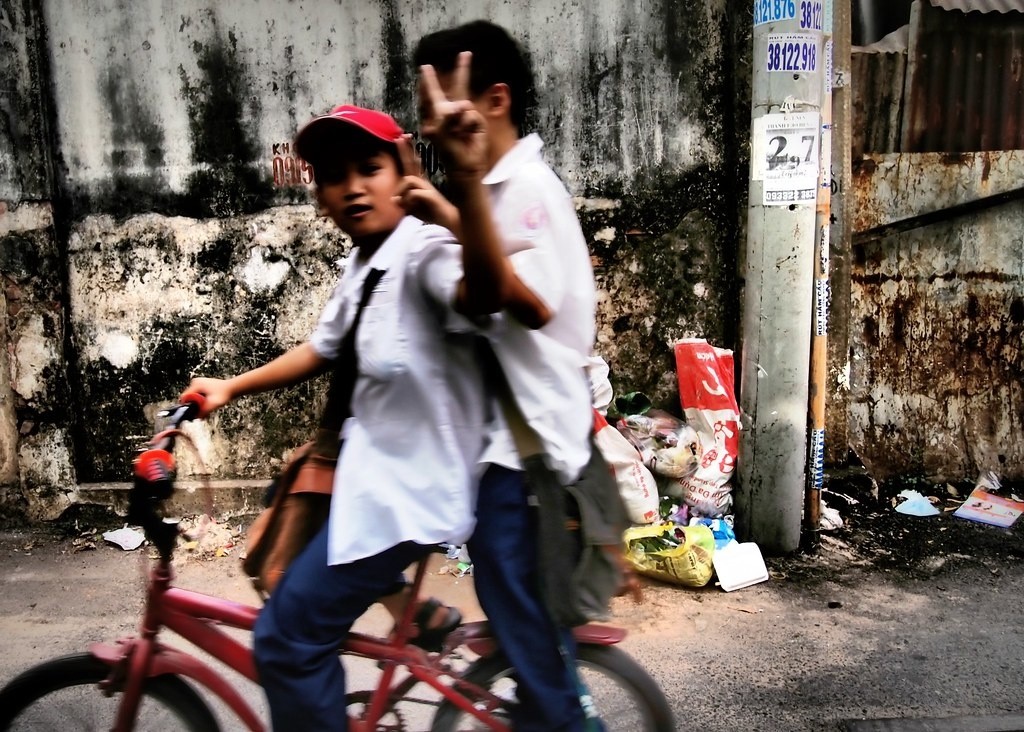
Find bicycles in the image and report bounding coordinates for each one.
[0,391,680,732]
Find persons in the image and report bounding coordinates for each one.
[177,43,554,732]
[388,19,611,732]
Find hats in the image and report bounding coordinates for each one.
[294,105,404,165]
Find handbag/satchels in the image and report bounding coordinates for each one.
[240,439,336,597]
[538,451,629,626]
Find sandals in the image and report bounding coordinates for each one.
[376,599,462,671]
